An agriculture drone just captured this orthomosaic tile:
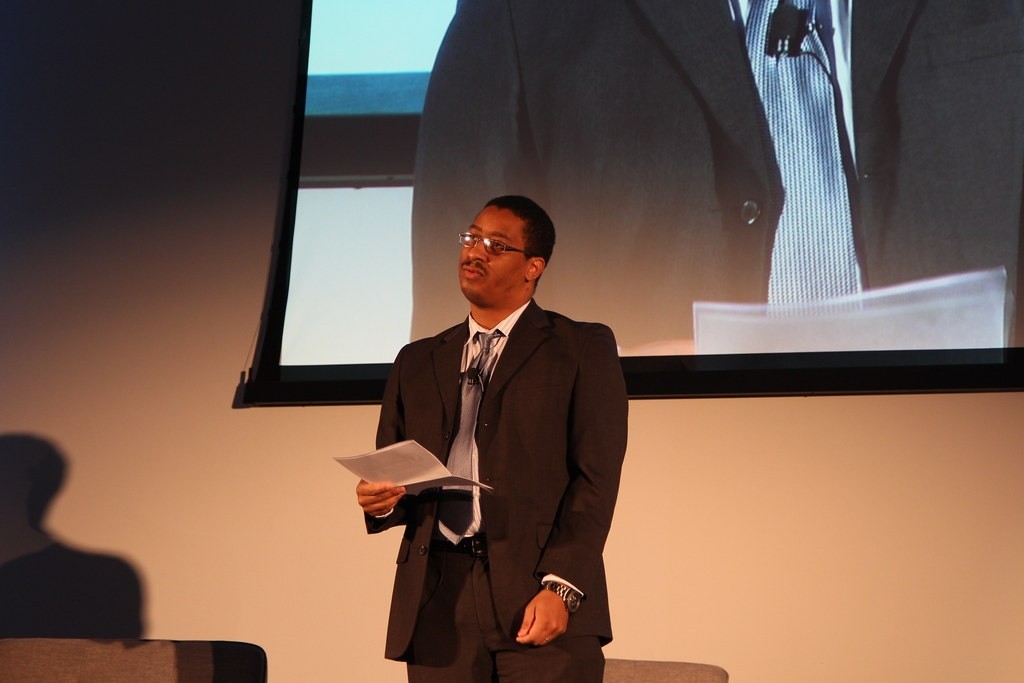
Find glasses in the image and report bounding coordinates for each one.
[458,233,532,256]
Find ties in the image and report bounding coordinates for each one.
[438,333,504,535]
[745,0,865,320]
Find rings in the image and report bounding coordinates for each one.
[546,638,548,640]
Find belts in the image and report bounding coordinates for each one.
[441,538,487,557]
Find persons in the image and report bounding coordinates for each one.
[412,0,1024,356]
[357,194,629,683]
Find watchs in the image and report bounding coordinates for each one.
[544,581,581,613]
